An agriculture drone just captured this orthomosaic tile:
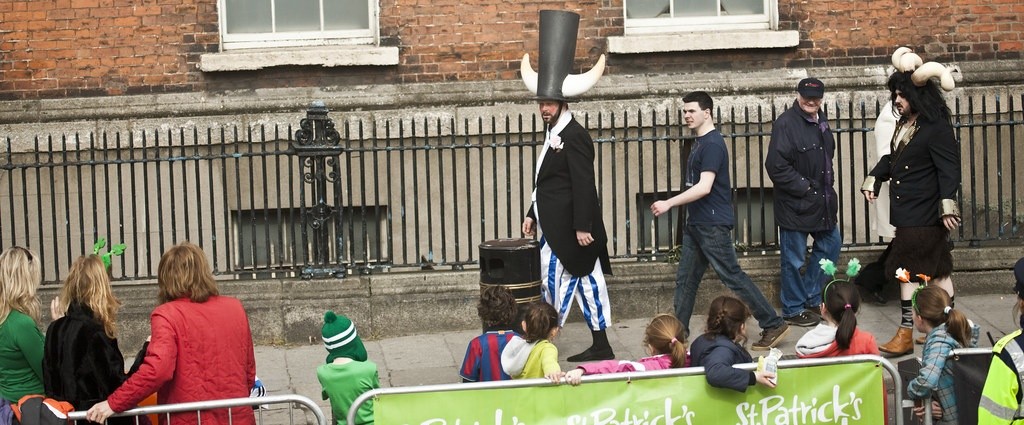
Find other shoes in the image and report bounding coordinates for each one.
[567,346,615,361]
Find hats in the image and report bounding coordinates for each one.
[1013,258,1024,300]
[321,311,368,363]
[798,79,824,97]
[521,9,606,103]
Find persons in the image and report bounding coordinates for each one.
[42,236,152,425]
[764,77,843,328]
[0,245,68,425]
[86,241,256,425]
[859,46,963,355]
[520,9,616,362]
[977,257,1024,425]
[689,295,776,393]
[460,285,521,383]
[316,311,380,425]
[564,313,690,387]
[907,284,981,425]
[794,257,888,425]
[500,302,566,386]
[650,90,791,351]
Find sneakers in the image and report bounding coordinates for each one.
[752,320,791,350]
[783,310,820,326]
[806,306,824,318]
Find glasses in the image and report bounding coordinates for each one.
[11,246,32,265]
[894,93,905,98]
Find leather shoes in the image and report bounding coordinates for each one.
[916,337,927,343]
[878,327,914,355]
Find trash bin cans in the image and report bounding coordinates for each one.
[478,237,543,335]
